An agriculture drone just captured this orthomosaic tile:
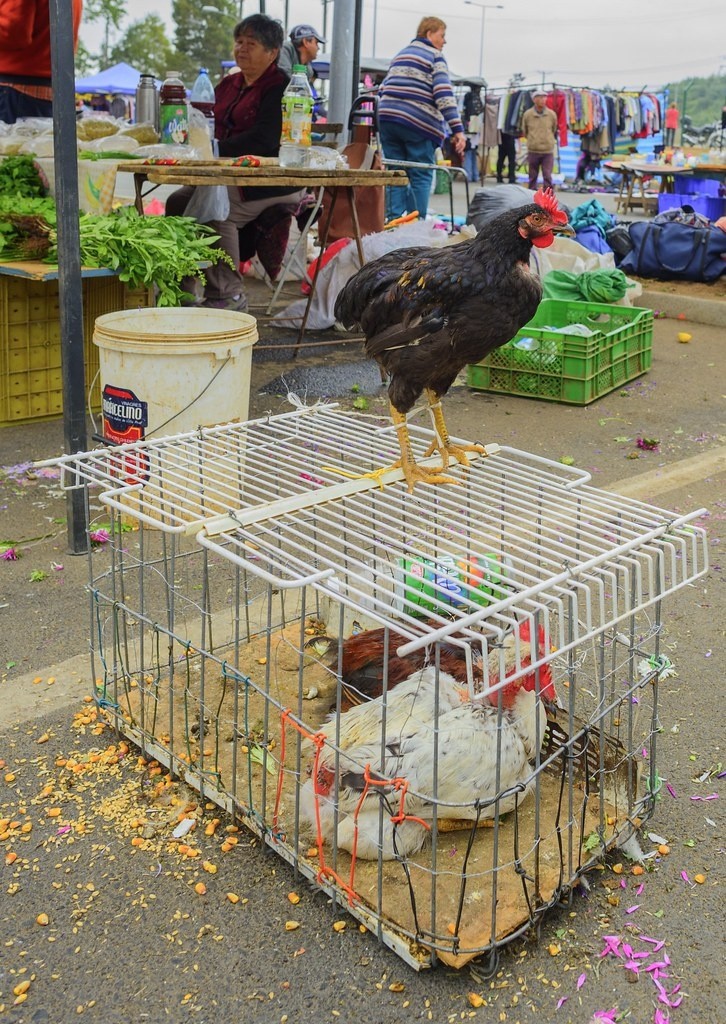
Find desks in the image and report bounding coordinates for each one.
[604,162,726,216]
[32,157,408,355]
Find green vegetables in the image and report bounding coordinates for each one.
[0,151,237,307]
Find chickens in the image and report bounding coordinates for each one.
[331,623,504,712]
[299,618,563,862]
[334,187,568,492]
[303,616,500,699]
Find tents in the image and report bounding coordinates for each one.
[76,62,191,98]
[220,57,487,187]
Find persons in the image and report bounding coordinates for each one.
[76,94,127,120]
[0,0,83,124]
[523,91,557,195]
[497,129,518,184]
[278,24,328,121]
[463,138,479,182]
[378,17,467,222]
[164,14,306,313]
[666,103,678,146]
[721,106,726,129]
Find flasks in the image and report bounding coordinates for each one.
[135,74,158,133]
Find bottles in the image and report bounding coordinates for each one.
[158,71,189,145]
[325,551,519,619]
[277,64,313,169]
[191,67,216,157]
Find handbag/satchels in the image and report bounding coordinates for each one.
[618,220,726,285]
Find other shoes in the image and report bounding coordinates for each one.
[194,293,248,314]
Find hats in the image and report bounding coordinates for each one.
[290,24,325,44]
[531,91,548,101]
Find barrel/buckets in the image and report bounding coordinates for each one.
[87,306,260,531]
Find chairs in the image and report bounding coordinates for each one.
[250,123,343,315]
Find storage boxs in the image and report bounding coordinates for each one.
[658,174,726,221]
[0,273,153,429]
[434,170,453,194]
[468,298,653,406]
